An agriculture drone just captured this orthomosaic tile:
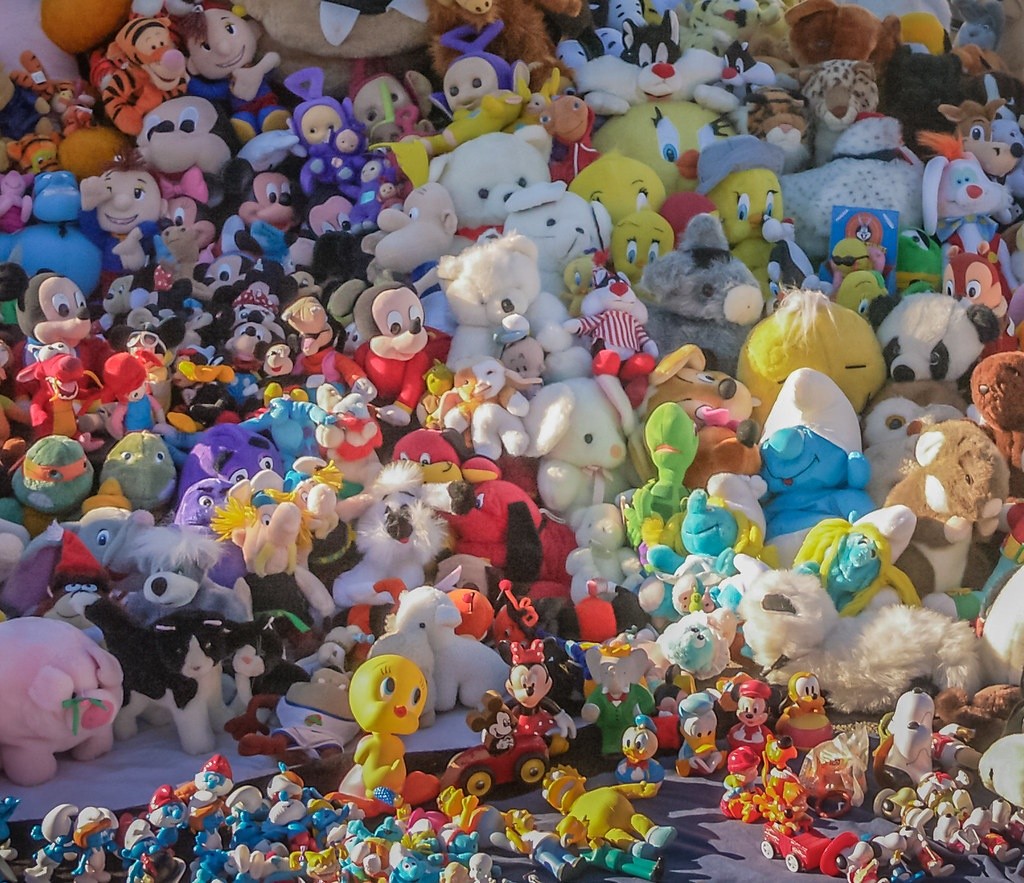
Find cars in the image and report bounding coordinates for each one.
[760,818,835,874]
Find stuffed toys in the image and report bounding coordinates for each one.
[0,0,1024,883]
